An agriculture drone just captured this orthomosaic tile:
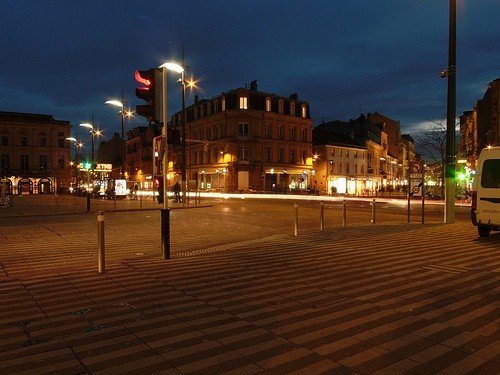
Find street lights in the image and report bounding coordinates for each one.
[66,137,80,190]
[159,42,190,204]
[104,98,127,178]
[77,113,94,178]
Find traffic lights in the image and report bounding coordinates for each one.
[133,68,157,121]
[83,162,93,170]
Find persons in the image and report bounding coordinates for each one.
[173,181,181,203]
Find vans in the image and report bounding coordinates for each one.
[471,146,500,236]
